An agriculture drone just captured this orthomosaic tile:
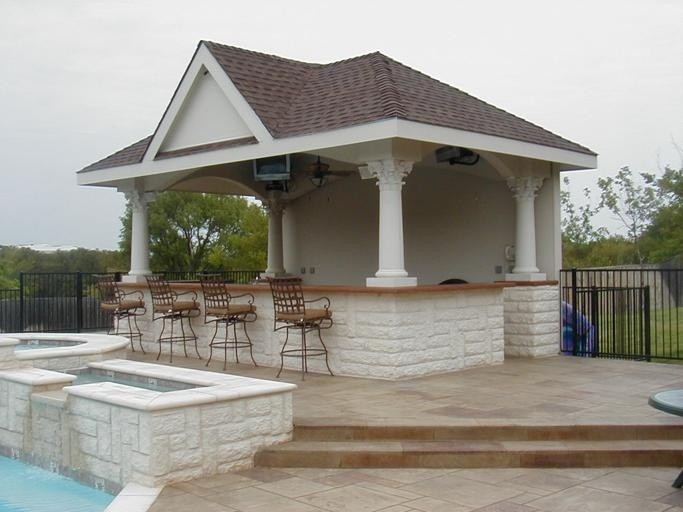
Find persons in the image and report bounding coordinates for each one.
[561,298,600,357]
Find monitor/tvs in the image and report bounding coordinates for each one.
[253,155,291,180]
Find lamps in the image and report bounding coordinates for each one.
[303,153,332,203]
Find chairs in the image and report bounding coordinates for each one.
[266,276,334,381]
[196,273,259,371]
[90,272,149,354]
[142,273,202,363]
